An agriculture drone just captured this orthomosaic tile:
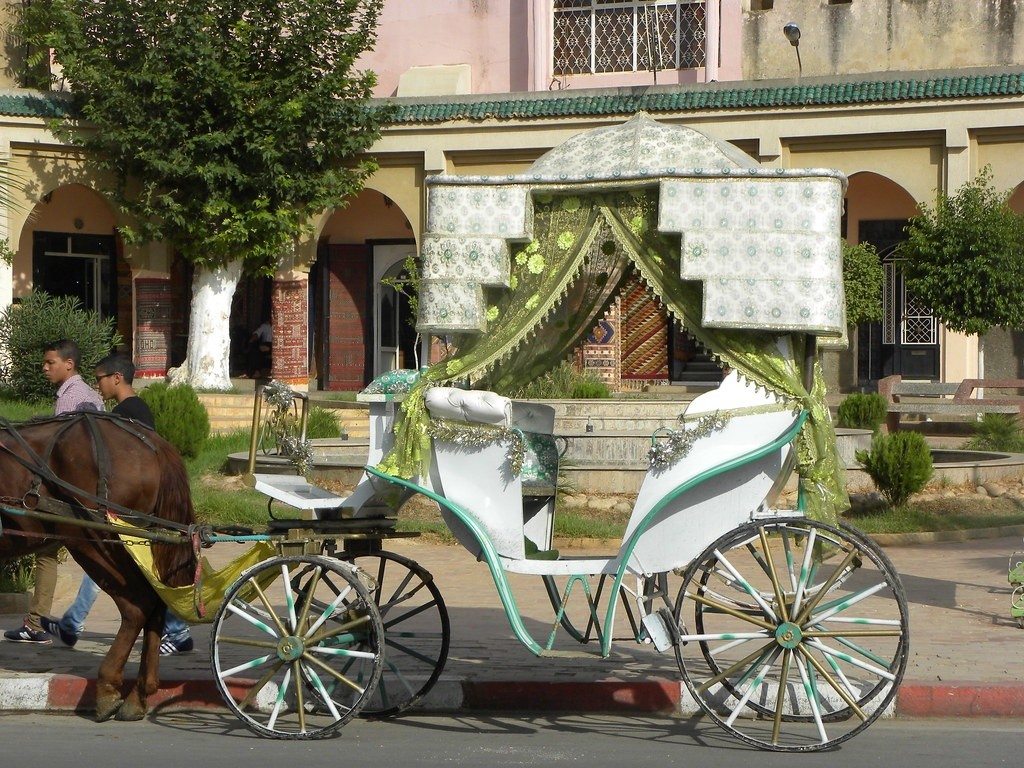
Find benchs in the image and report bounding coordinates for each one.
[425,386,559,497]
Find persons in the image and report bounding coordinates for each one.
[4,339,105,645]
[248,314,272,379]
[40,352,195,658]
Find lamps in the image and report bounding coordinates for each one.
[784,22,802,77]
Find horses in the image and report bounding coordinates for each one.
[0,410,197,722]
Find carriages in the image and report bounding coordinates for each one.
[0,107,914,756]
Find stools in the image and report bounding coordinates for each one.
[356,369,422,404]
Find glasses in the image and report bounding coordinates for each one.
[96,373,114,383]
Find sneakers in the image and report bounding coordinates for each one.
[39,614,79,648]
[159,625,193,654]
[3,619,53,644]
